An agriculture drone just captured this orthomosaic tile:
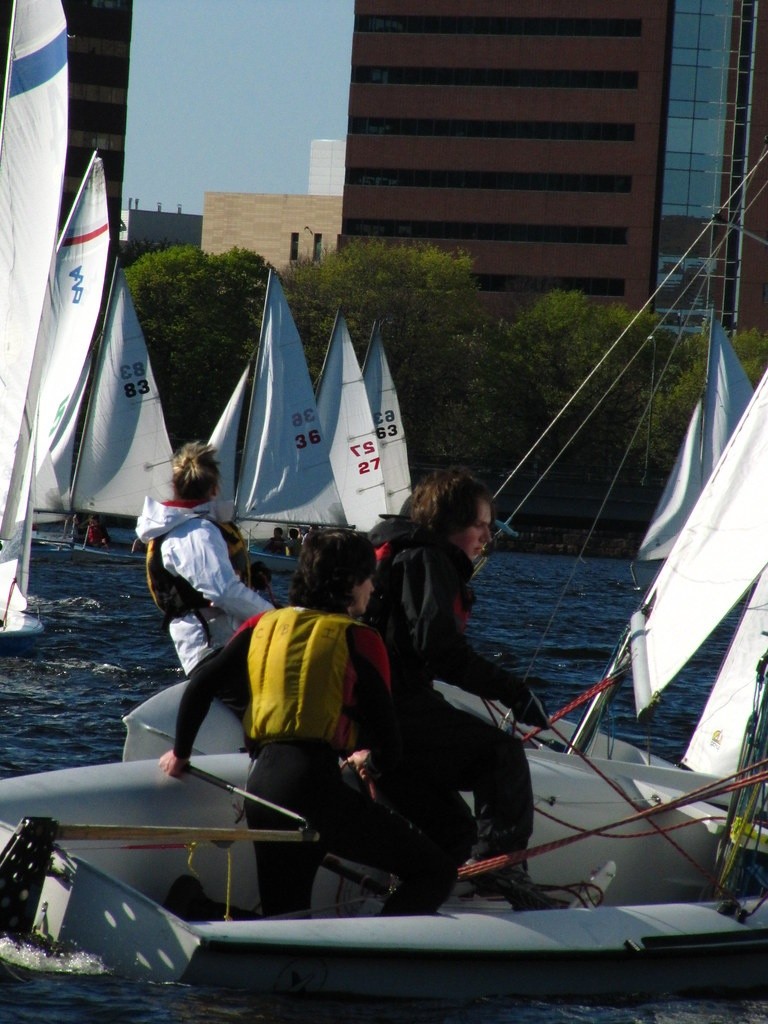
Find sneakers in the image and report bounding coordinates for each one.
[463,849,560,911]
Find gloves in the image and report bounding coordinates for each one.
[497,670,549,729]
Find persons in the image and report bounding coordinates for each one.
[87,515,113,551]
[301,525,319,545]
[135,442,275,718]
[73,513,86,542]
[264,528,285,554]
[285,529,301,557]
[158,527,456,917]
[348,470,555,911]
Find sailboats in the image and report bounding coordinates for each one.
[0,0,768,999]
